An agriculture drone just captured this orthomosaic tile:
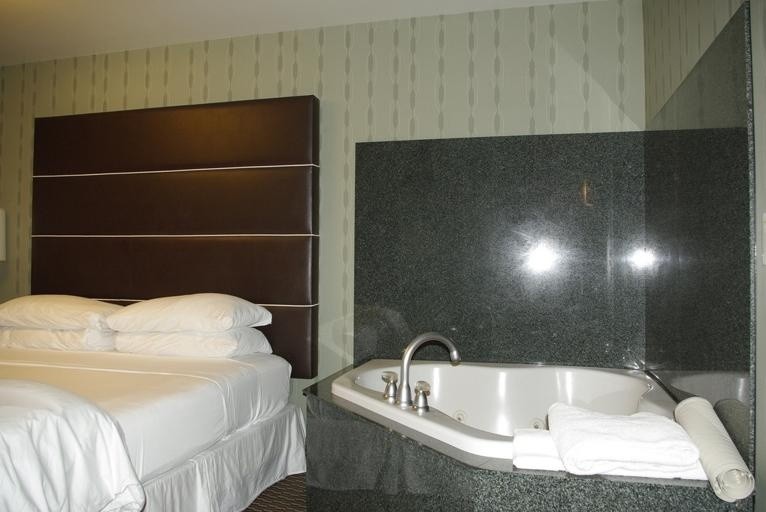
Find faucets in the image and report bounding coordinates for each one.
[396,330,462,409]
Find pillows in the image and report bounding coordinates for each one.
[0,328,105,354]
[0,293,124,330]
[103,292,273,335]
[105,329,273,362]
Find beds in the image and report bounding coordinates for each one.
[0,94,319,512]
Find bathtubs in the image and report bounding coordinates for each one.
[670,371,749,414]
[345,365,695,466]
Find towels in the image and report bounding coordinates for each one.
[512,427,566,472]
[547,402,707,481]
[673,395,755,504]
